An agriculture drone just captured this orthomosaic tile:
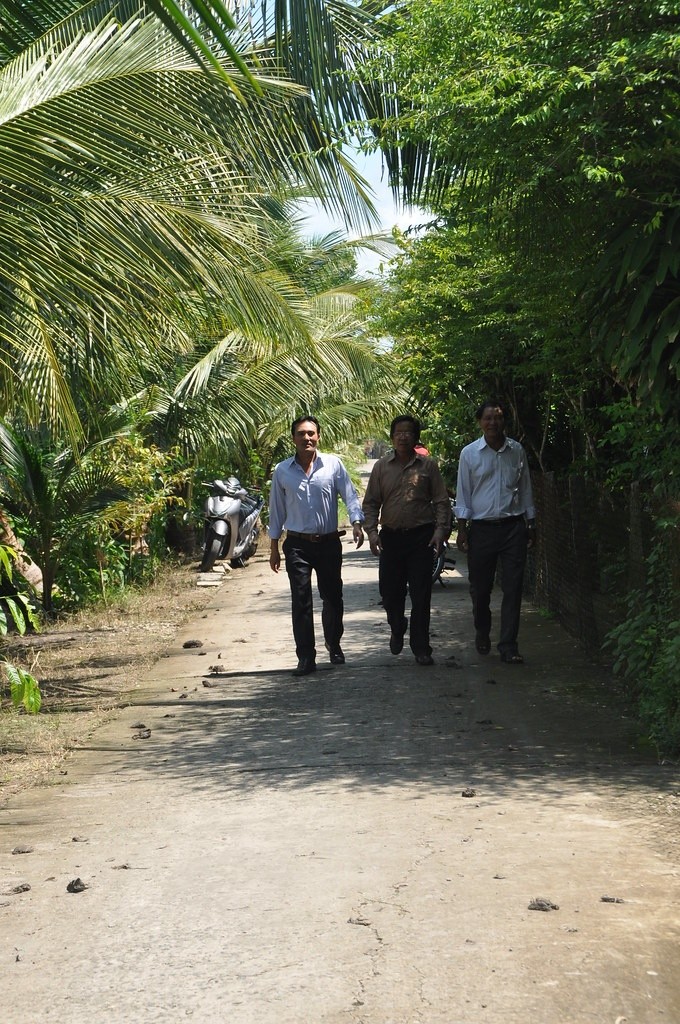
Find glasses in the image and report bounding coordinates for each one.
[393,432,417,439]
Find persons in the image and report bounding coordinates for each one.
[453,401,538,664]
[362,412,454,666]
[268,416,365,675]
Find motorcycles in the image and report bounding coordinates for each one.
[405,539,456,592]
[199,478,265,573]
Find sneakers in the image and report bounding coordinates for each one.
[292,655,316,675]
[325,639,345,664]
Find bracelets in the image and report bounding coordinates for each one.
[528,524,537,529]
[354,520,362,527]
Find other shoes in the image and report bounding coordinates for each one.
[500,648,524,664]
[416,652,433,665]
[475,629,491,655]
[389,617,408,655]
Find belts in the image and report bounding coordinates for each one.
[472,515,522,528]
[286,530,346,543]
[382,523,434,537]
